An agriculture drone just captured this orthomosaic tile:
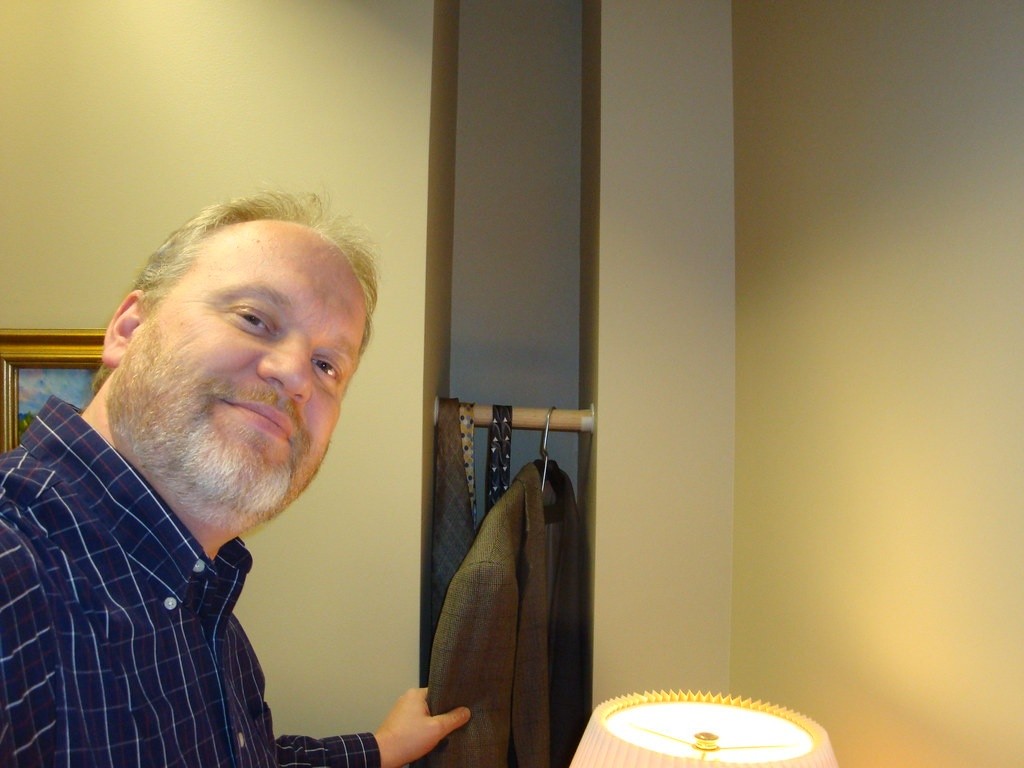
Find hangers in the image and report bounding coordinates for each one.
[542,406,556,489]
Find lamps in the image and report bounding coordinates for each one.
[568,689,839,768]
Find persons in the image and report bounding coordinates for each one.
[0,191,471,768]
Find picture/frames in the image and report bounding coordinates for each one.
[0,327,107,456]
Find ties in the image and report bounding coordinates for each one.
[488,405,512,510]
[459,402,477,537]
[430,398,475,635]
